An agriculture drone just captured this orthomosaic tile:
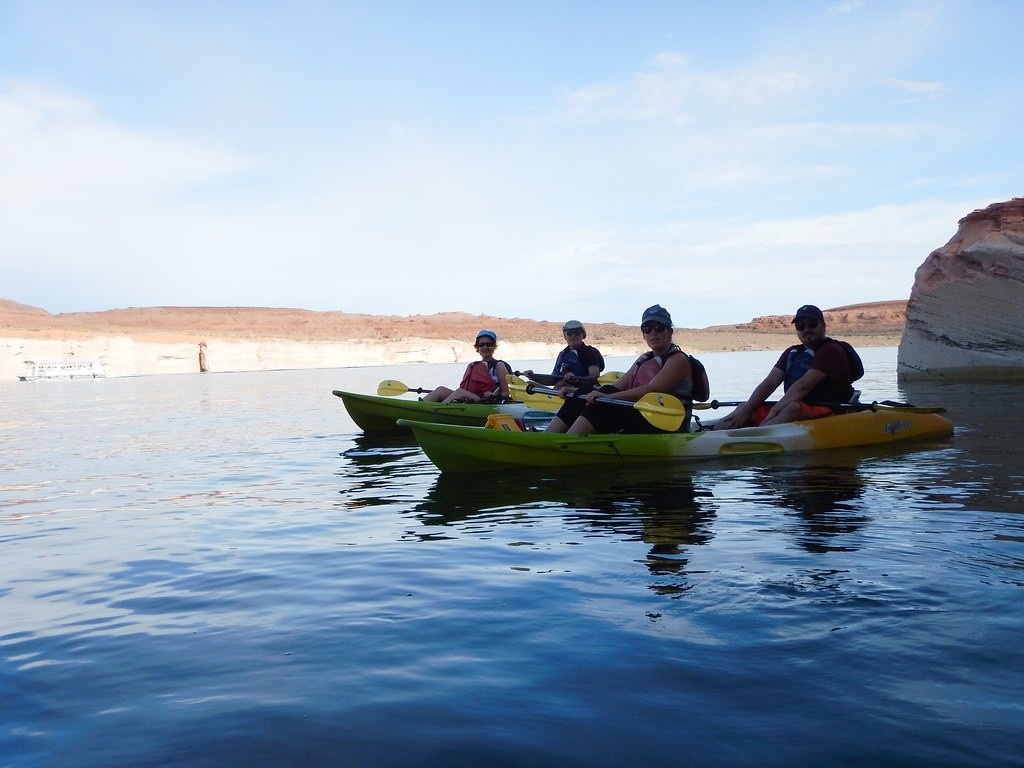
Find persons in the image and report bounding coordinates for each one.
[710,304,864,431]
[420,329,514,402]
[541,305,693,434]
[550,320,605,393]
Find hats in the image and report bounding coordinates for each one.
[791,305,822,323]
[641,305,671,327]
[562,320,586,334]
[477,330,496,341]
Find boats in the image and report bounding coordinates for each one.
[332,389,559,438]
[395,400,954,474]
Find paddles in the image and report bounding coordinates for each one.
[376,367,948,444]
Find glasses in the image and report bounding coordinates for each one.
[477,343,495,347]
[566,331,583,335]
[794,320,819,329]
[642,325,668,334]
